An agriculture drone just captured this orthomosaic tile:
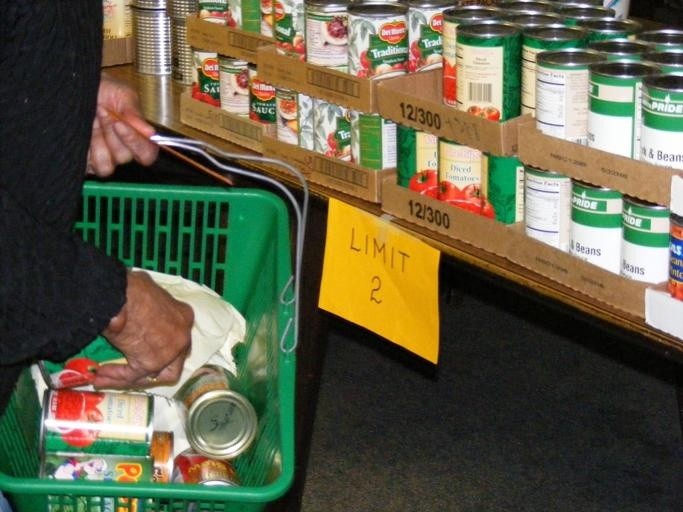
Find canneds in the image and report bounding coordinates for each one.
[349,5,410,83]
[174,361,260,460]
[481,152,525,229]
[587,66,662,162]
[457,21,521,122]
[399,0,458,74]
[248,68,277,125]
[348,110,396,182]
[437,137,483,197]
[312,100,353,163]
[229,0,276,35]
[622,194,670,284]
[516,17,574,29]
[580,18,645,42]
[39,450,157,505]
[503,2,555,16]
[171,452,242,488]
[571,179,620,275]
[37,390,152,452]
[197,0,229,26]
[590,43,655,58]
[641,76,683,169]
[274,89,298,143]
[520,166,571,255]
[296,93,318,154]
[643,29,683,54]
[668,215,683,302]
[395,125,434,187]
[272,0,306,59]
[33,334,126,389]
[534,48,606,146]
[190,48,220,107]
[522,27,585,119]
[305,3,349,70]
[639,51,683,74]
[216,55,250,117]
[441,5,503,108]
[559,3,619,17]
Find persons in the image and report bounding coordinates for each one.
[0,0,196,512]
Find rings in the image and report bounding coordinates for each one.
[146,375,160,387]
